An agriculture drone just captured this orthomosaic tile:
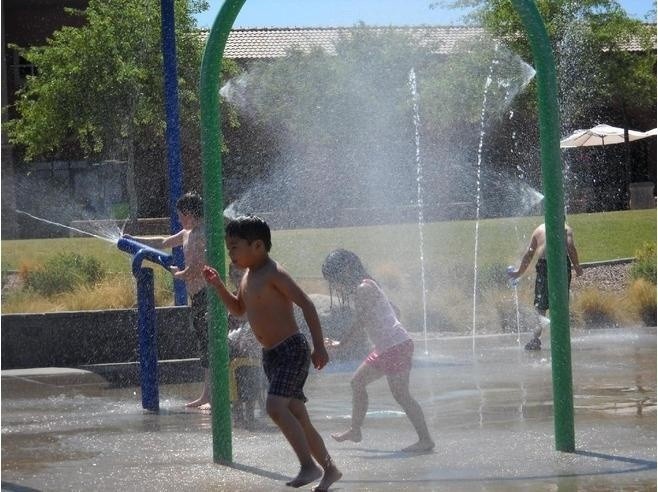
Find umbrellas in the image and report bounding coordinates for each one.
[558,122,657,210]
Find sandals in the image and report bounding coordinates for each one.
[524,338,542,351]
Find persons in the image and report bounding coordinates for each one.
[203,215,342,490]
[321,248,435,454]
[122,190,212,411]
[508,206,582,348]
[226,264,270,430]
